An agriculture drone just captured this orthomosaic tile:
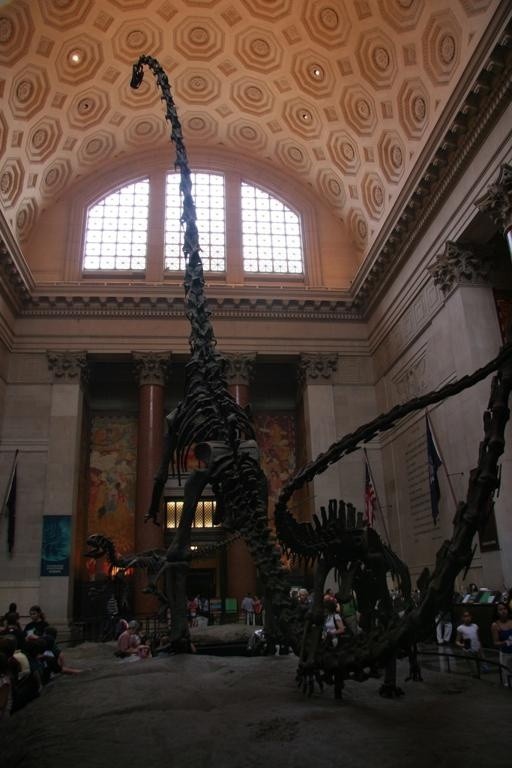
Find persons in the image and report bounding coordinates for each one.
[0,602,85,719]
[293,588,361,650]
[186,592,210,628]
[454,609,490,680]
[490,603,512,689]
[439,615,453,644]
[389,584,421,618]
[114,618,153,663]
[241,591,267,626]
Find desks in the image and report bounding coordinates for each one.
[452,602,499,647]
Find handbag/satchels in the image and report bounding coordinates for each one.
[195,606,200,614]
[335,628,353,648]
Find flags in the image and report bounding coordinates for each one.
[420,409,444,525]
[6,464,17,553]
[361,457,378,530]
